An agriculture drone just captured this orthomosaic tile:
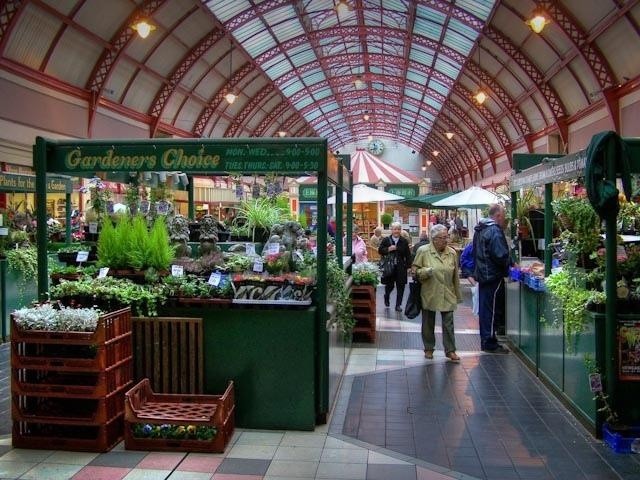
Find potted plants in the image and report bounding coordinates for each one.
[480,178,640,366]
[380,212,394,231]
[584,351,636,438]
[0,192,379,337]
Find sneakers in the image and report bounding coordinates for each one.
[384,295,390,307]
[449,352,461,362]
[395,305,402,312]
[425,350,434,359]
[484,347,510,354]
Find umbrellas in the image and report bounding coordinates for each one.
[420,188,491,224]
[432,184,511,225]
[327,182,407,232]
[400,191,436,221]
[294,147,424,184]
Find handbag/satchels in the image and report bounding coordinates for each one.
[380,261,406,286]
[404,281,422,320]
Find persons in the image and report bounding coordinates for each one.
[503,218,512,257]
[430,212,466,232]
[460,242,480,325]
[409,224,462,360]
[14,207,86,243]
[408,232,431,260]
[475,203,513,356]
[341,225,368,261]
[378,223,411,312]
[369,227,383,257]
[308,216,334,237]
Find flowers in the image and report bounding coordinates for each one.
[130,422,217,441]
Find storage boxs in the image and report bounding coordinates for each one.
[124,377,236,426]
[11,306,135,453]
[602,423,639,455]
[124,404,236,453]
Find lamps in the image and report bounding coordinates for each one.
[425,136,433,166]
[443,85,456,140]
[362,92,370,123]
[332,0,354,22]
[224,35,239,104]
[366,121,374,142]
[524,1,551,36]
[471,33,491,106]
[421,150,428,171]
[278,93,287,138]
[128,1,159,41]
[431,119,440,156]
[355,31,366,94]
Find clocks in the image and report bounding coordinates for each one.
[367,138,385,157]
